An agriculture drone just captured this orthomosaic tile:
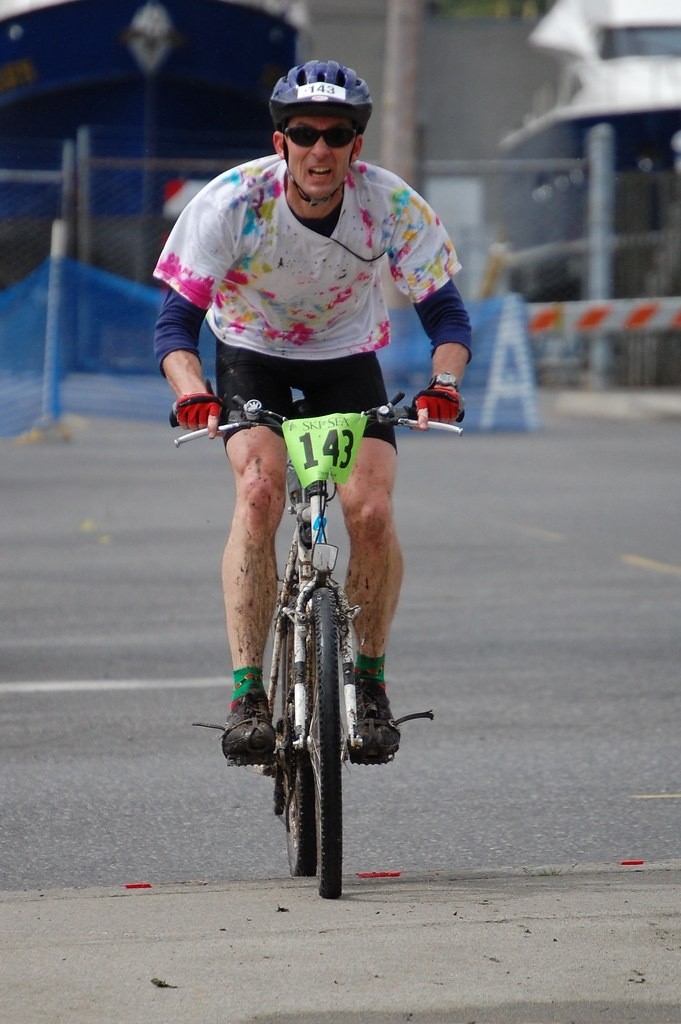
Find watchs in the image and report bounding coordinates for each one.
[430,371,460,392]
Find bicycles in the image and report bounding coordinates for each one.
[167,393,466,898]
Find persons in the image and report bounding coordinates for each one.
[150,52,478,768]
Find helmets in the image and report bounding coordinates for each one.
[268,60,372,130]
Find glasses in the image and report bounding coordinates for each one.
[283,126,357,148]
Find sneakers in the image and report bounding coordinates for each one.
[221,693,276,755]
[347,677,401,760]
